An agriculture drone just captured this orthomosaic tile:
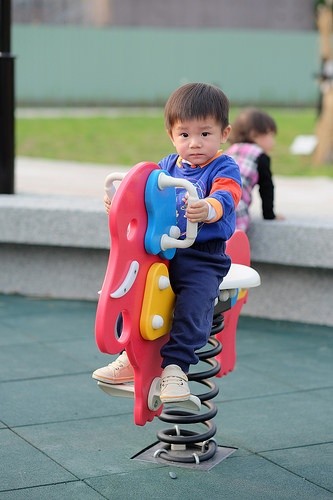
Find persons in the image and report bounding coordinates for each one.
[224,108,286,231]
[92,81,244,404]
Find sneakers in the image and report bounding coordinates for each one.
[159,364,190,403]
[92,351,134,384]
